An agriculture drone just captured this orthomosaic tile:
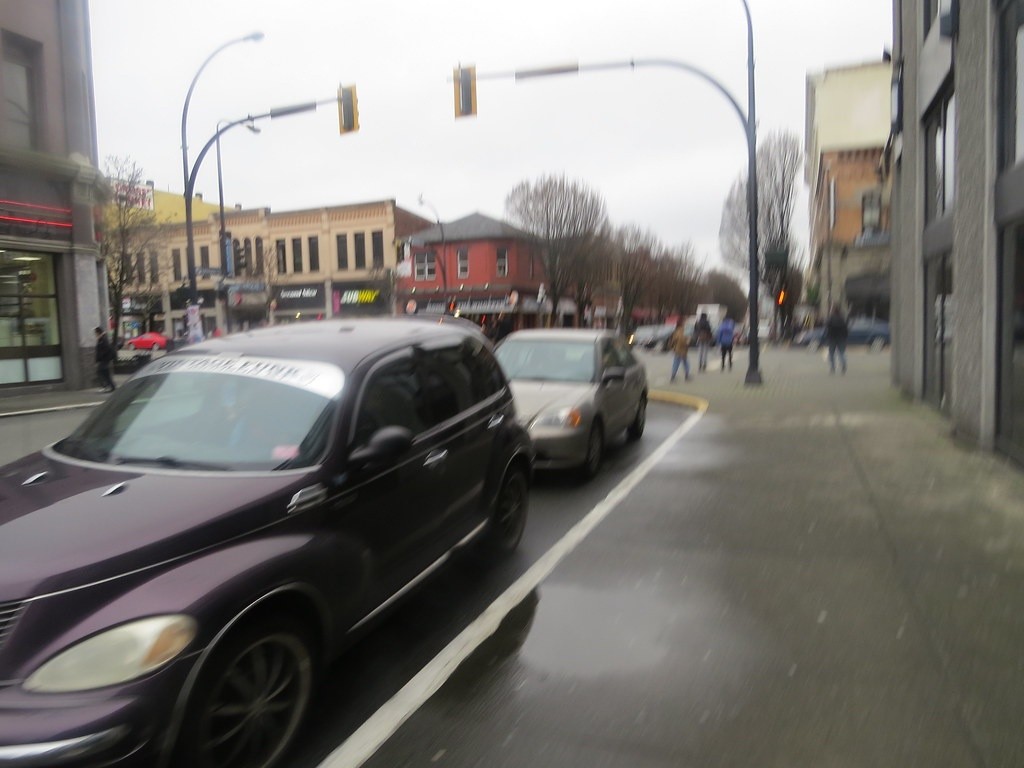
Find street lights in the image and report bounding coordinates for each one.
[214,117,261,275]
[182,30,268,301]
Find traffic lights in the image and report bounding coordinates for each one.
[776,280,788,307]
[232,242,247,277]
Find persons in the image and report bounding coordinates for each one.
[826,309,848,374]
[671,319,690,380]
[694,313,712,370]
[94,327,115,393]
[717,317,735,370]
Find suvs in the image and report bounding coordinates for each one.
[0,312,534,768]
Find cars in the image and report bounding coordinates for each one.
[126,332,169,351]
[793,312,891,354]
[492,327,649,484]
[628,302,787,352]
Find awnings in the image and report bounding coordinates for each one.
[238,310,264,320]
